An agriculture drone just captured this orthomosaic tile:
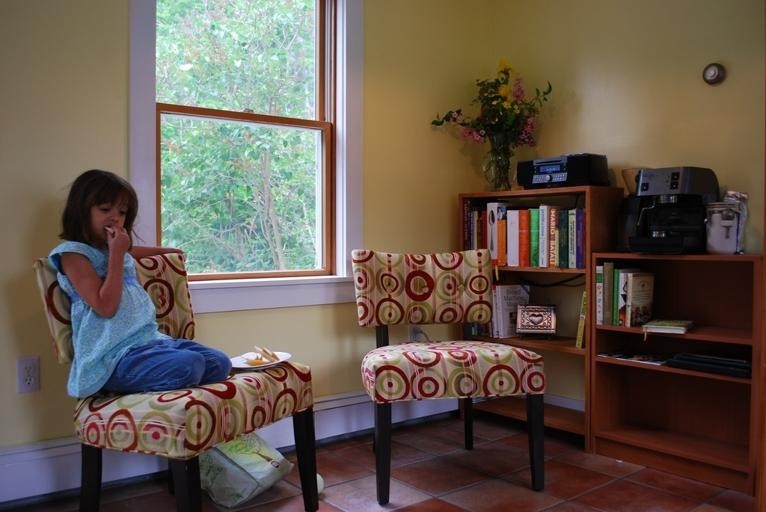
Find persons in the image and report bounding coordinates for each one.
[48,169,232,400]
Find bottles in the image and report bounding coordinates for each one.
[705,200,736,254]
[721,190,750,254]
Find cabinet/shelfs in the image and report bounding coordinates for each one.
[459,186,623,456]
[589,250,766,498]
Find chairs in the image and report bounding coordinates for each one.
[31,244,321,511]
[350,248,549,506]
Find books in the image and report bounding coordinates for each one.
[594,262,751,374]
[463,200,588,349]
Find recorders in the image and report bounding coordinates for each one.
[517,153,611,189]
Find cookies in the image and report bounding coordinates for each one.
[103,226,114,233]
[241,345,279,367]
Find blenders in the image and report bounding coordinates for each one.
[612,169,640,249]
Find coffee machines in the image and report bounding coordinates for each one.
[628,165,720,256]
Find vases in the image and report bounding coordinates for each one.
[482,148,512,191]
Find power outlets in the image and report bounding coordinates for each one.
[15,356,41,395]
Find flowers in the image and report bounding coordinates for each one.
[430,53,552,184]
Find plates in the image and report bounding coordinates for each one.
[228,350,294,371]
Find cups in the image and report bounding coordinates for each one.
[465,323,487,339]
